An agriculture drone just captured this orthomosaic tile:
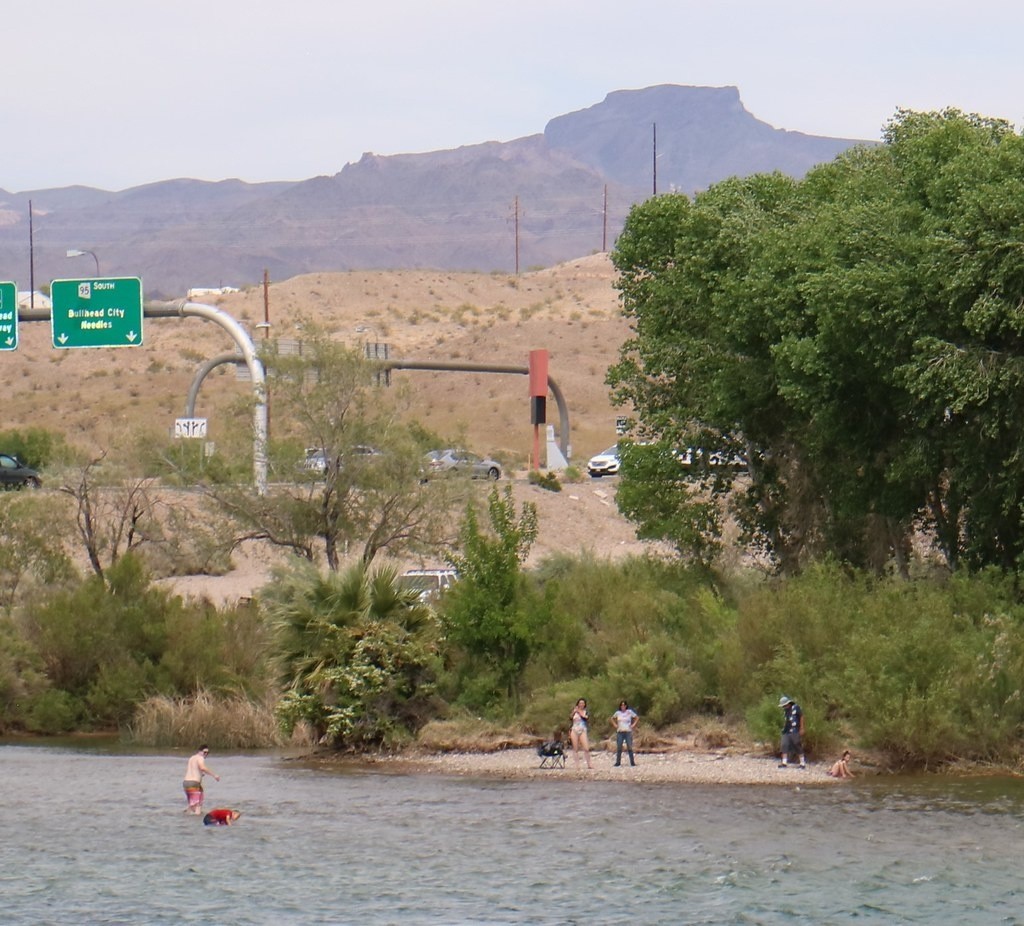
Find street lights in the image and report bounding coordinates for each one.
[67,250,100,278]
[356,325,378,359]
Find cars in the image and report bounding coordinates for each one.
[587,443,649,478]
[420,449,501,485]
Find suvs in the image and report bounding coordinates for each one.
[0,454,40,490]
[297,446,375,479]
[675,435,749,474]
[394,569,457,610]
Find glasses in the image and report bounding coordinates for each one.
[203,750,209,754]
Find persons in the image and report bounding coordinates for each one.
[203,809,241,825]
[611,700,640,766]
[777,696,806,771]
[831,751,855,778]
[569,697,594,769]
[182,744,220,813]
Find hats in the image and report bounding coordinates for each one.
[778,697,793,707]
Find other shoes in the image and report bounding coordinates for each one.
[632,763,637,766]
[615,762,620,766]
[797,765,805,769]
[778,763,787,768]
